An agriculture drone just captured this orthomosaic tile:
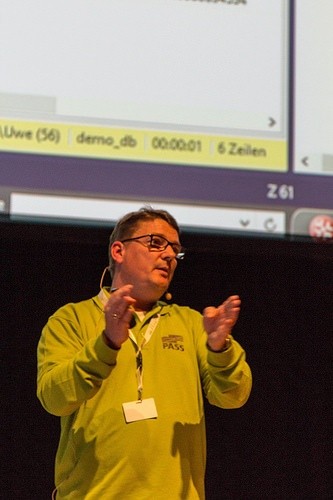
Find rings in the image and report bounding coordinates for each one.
[111,313,121,321]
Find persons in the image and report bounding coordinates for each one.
[37,211,251,500]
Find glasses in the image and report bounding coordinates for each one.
[120,234,186,260]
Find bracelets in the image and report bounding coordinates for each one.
[103,332,121,350]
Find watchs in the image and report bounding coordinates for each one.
[206,337,230,352]
